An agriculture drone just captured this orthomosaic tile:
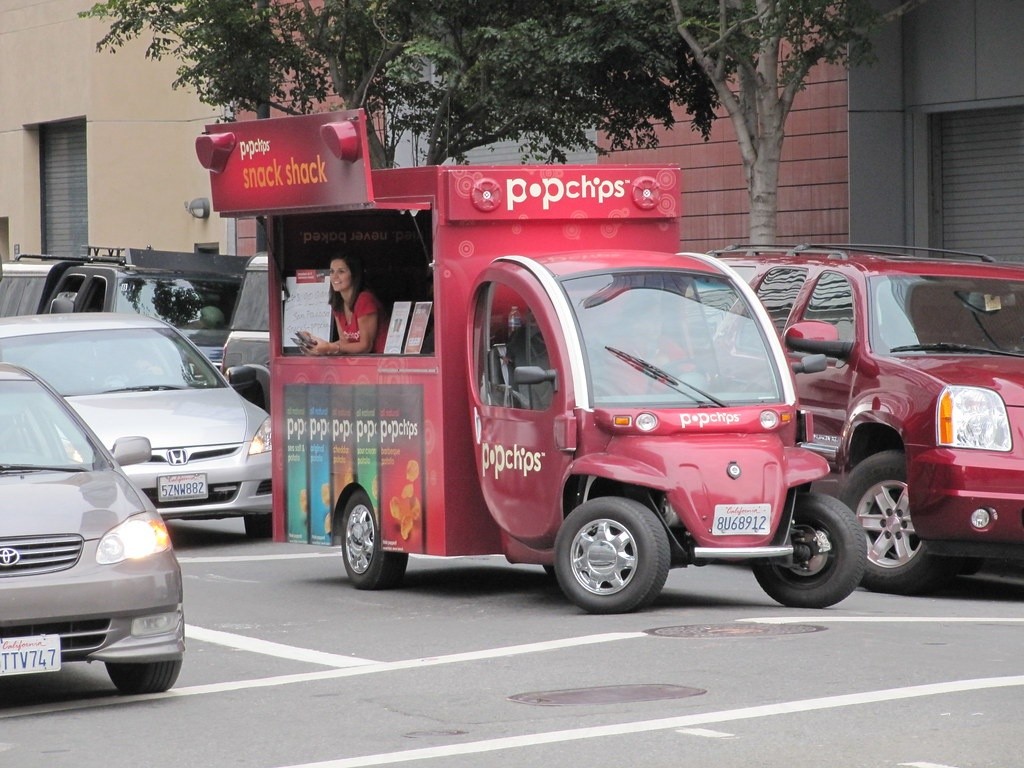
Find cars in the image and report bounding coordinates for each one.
[0,361,185,692]
[0,311,272,539]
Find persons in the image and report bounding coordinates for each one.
[606,289,710,395]
[291,252,389,357]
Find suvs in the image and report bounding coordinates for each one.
[222,253,273,414]
[685,241,1024,592]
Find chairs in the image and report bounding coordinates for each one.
[507,324,609,410]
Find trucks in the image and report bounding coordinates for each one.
[0,242,252,382]
[195,106,867,615]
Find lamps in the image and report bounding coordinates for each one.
[189,198,210,218]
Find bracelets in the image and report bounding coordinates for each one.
[332,344,340,356]
